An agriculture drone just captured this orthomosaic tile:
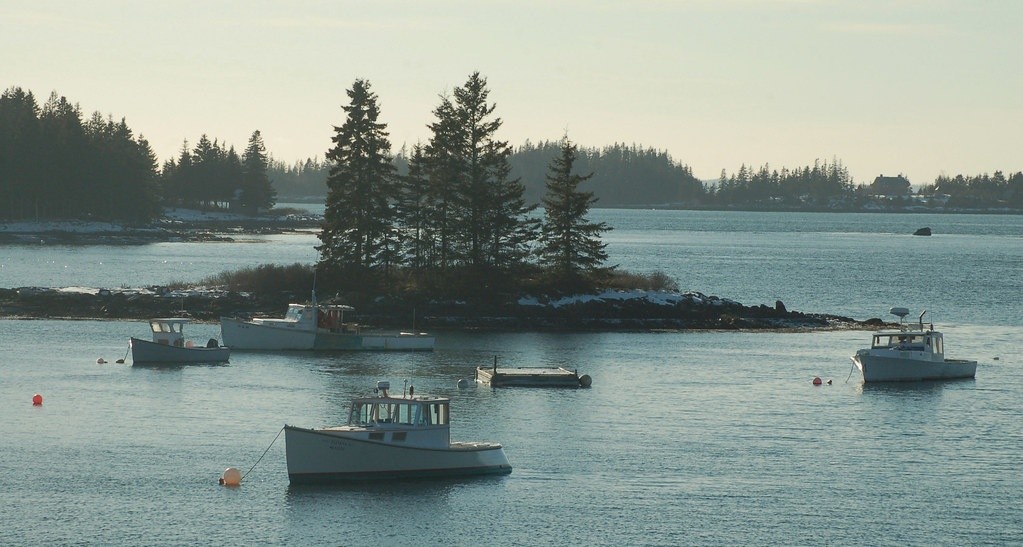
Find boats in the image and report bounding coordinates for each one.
[219,242,435,352]
[131,299,230,364]
[850,307,977,382]
[284,308,513,487]
